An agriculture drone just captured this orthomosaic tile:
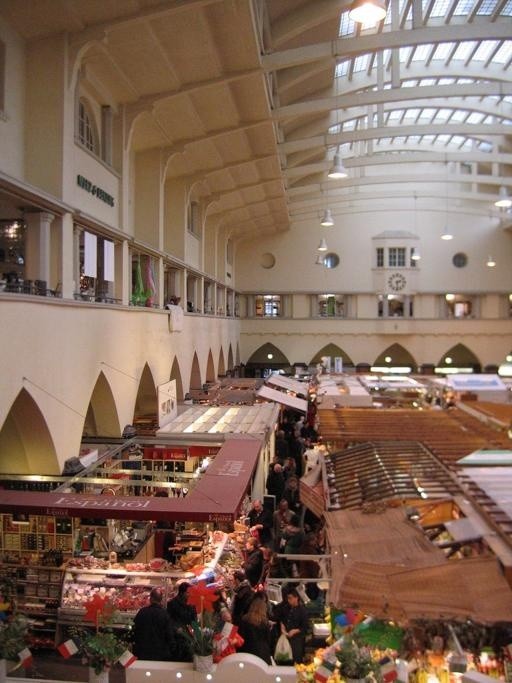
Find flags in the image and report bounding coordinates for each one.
[145,255,158,307]
[57,638,79,660]
[118,650,138,668]
[133,253,145,298]
[17,648,34,670]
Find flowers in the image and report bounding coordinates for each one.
[177,578,219,654]
[68,593,128,676]
[334,608,375,678]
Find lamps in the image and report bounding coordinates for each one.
[439,157,454,240]
[327,55,348,179]
[315,162,335,264]
[348,0,387,25]
[410,195,422,260]
[493,135,512,207]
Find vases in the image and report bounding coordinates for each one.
[192,653,213,673]
[344,675,367,683]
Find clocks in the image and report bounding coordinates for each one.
[388,272,406,290]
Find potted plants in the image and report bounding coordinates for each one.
[0,611,33,682]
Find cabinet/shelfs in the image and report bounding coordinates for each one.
[0,510,80,658]
[81,443,227,567]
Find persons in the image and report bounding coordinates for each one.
[133,589,177,662]
[266,456,299,515]
[198,567,232,629]
[17,557,29,594]
[167,582,198,633]
[241,499,326,601]
[213,620,244,663]
[275,380,323,476]
[238,589,308,666]
[232,572,256,626]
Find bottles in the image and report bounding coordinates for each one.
[154,460,163,472]
